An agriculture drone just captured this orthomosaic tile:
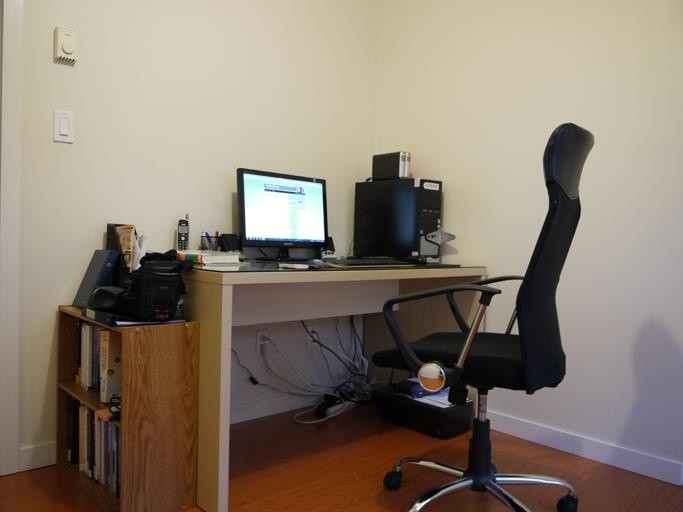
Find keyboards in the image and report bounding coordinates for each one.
[314,257,416,268]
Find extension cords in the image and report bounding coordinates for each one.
[327,401,356,416]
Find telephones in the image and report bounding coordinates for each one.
[177,213,189,251]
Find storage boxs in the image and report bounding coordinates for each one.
[374,381,473,439]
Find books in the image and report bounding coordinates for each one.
[115,224,134,272]
[275,261,320,270]
[371,152,404,180]
[176,248,240,264]
[191,263,240,272]
[403,152,411,179]
[62,321,123,496]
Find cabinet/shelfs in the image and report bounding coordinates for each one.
[56,304,199,511]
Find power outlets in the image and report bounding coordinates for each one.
[256,329,271,355]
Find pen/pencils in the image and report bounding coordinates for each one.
[201,231,219,250]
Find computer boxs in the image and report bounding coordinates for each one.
[354,179,442,262]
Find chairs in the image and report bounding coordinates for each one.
[369,122,598,512]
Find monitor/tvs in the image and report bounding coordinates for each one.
[237,168,327,261]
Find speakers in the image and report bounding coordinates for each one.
[320,237,337,260]
[218,234,248,260]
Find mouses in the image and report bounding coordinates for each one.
[406,256,427,264]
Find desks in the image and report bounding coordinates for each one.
[176,266,486,511]
